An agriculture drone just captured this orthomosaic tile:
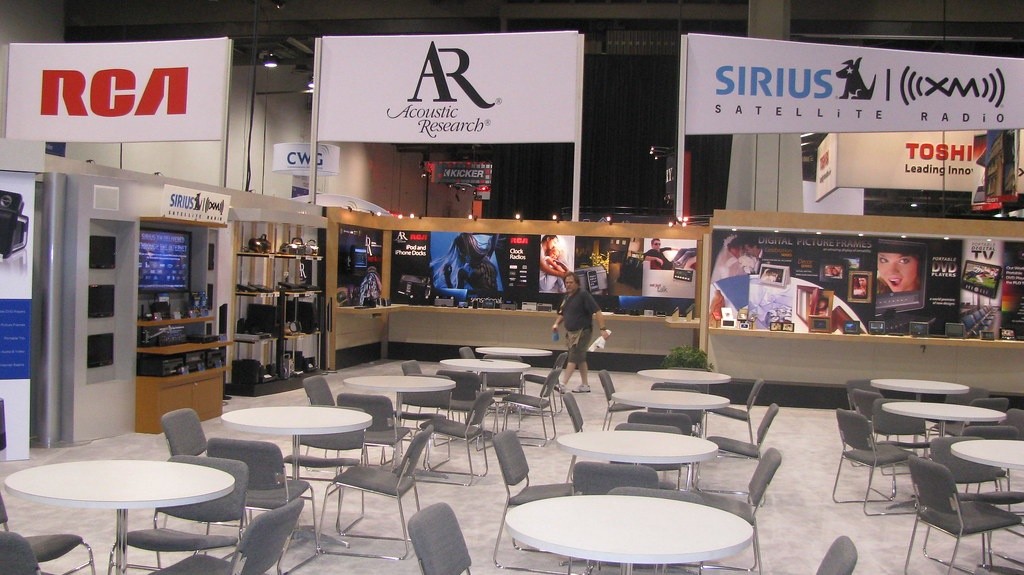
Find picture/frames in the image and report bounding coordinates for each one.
[842,320,860,334]
[819,258,848,284]
[868,321,885,335]
[808,315,832,333]
[945,323,964,339]
[782,323,794,332]
[758,264,787,287]
[847,270,873,304]
[909,321,929,337]
[979,330,994,341]
[806,291,834,322]
[770,322,782,331]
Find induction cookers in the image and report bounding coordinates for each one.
[278,280,321,292]
[235,281,275,294]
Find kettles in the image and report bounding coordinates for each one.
[249,233,271,253]
[280,238,319,255]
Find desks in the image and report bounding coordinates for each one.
[612,390,730,491]
[950,439,1024,471]
[475,347,553,414]
[220,406,373,548]
[922,435,1024,575]
[438,357,531,438]
[880,402,1007,498]
[4,459,235,575]
[343,375,457,480]
[869,379,969,453]
[505,496,754,575]
[557,430,718,568]
[636,370,733,385]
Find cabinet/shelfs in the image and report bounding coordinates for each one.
[222,208,328,398]
[135,316,233,435]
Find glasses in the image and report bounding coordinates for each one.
[655,243,660,245]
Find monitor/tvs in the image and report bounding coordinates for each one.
[962,260,1003,300]
[137,226,191,293]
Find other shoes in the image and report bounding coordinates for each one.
[572,385,591,393]
[554,385,565,394]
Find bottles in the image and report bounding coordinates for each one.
[552,328,560,342]
[190,289,209,317]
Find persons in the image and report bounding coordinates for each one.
[551,270,610,394]
[642,238,699,270]
[442,233,498,294]
[877,251,921,296]
[815,264,868,314]
[358,265,383,305]
[708,233,796,331]
[539,234,574,295]
[963,263,998,287]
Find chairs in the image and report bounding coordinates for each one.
[627,412,693,491]
[0,345,573,575]
[816,535,858,575]
[830,378,1024,517]
[610,423,683,490]
[572,461,659,495]
[673,446,782,575]
[703,378,765,460]
[648,387,706,484]
[651,382,709,394]
[597,369,645,430]
[563,391,583,483]
[693,403,779,507]
[904,454,1022,575]
[664,367,710,395]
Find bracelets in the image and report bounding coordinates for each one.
[600,326,607,331]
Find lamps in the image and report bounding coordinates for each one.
[263,55,278,68]
